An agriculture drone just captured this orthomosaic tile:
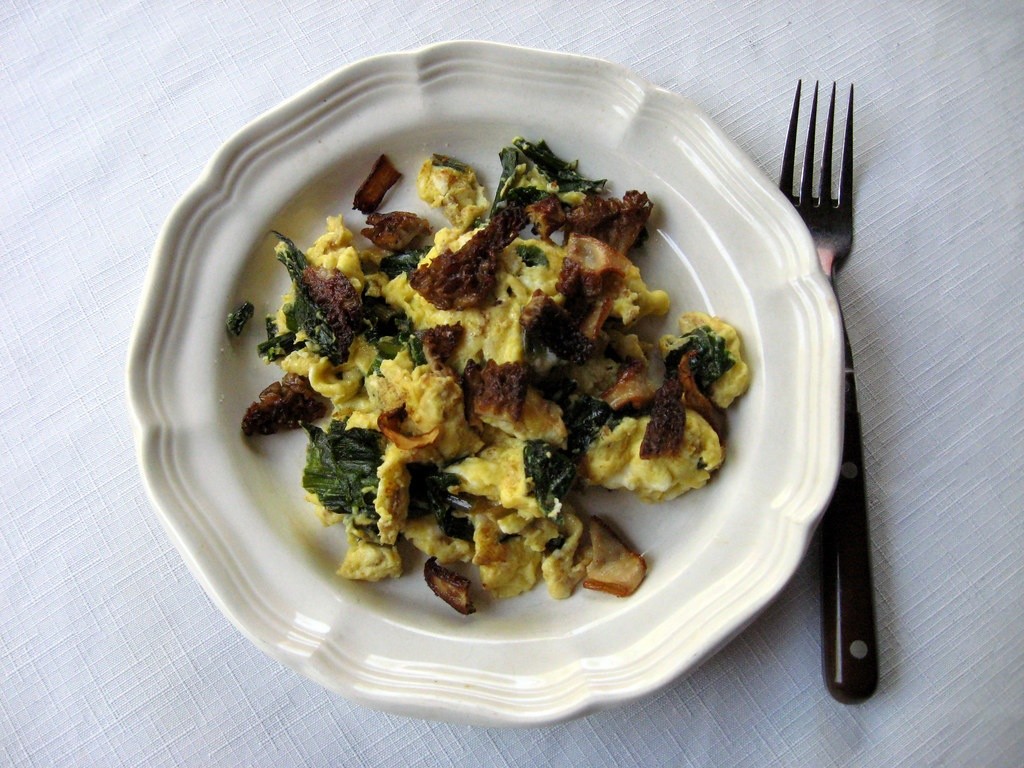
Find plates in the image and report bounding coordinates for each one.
[131,41,847,723]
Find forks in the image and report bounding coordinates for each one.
[778,77,879,703]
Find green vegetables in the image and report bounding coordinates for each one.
[227,140,734,536]
